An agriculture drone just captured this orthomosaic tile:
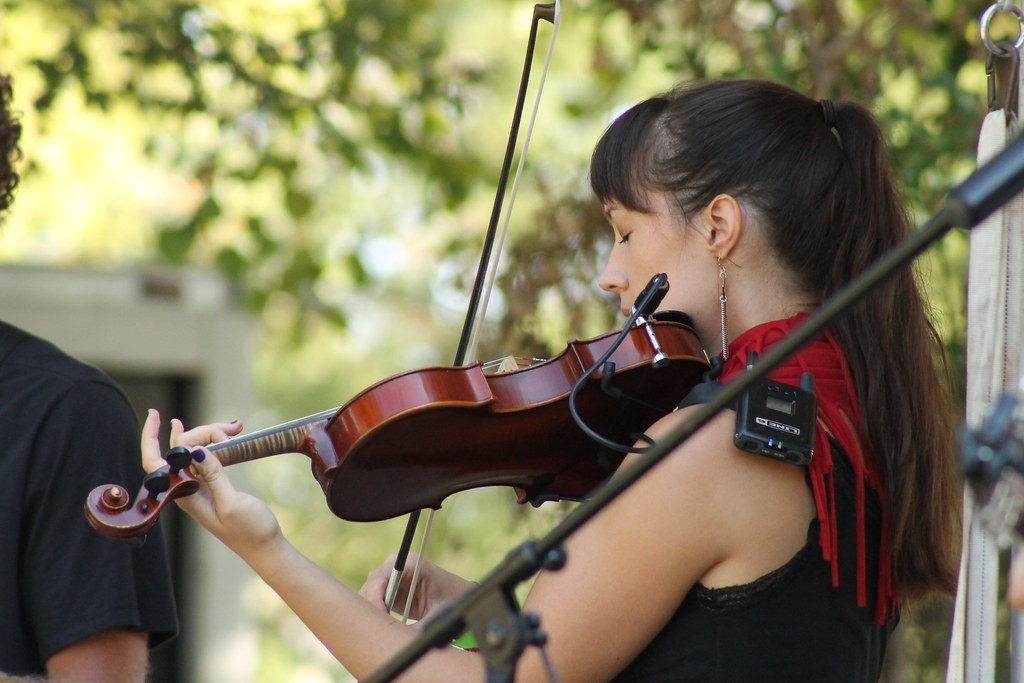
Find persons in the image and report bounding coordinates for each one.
[0,74,180,683]
[141,79,964,683]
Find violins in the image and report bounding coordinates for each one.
[82,317,708,539]
